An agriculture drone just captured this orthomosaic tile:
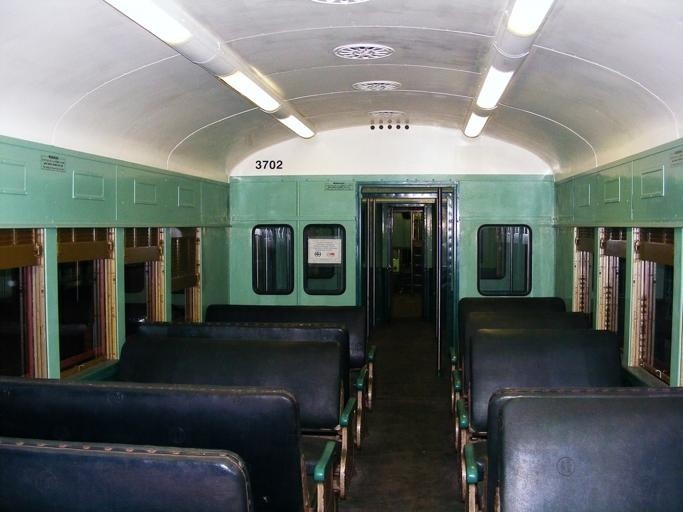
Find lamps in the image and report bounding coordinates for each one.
[102,0,318,142]
[461,0,558,141]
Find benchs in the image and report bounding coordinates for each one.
[450,297,683,510]
[1,299,379,512]
